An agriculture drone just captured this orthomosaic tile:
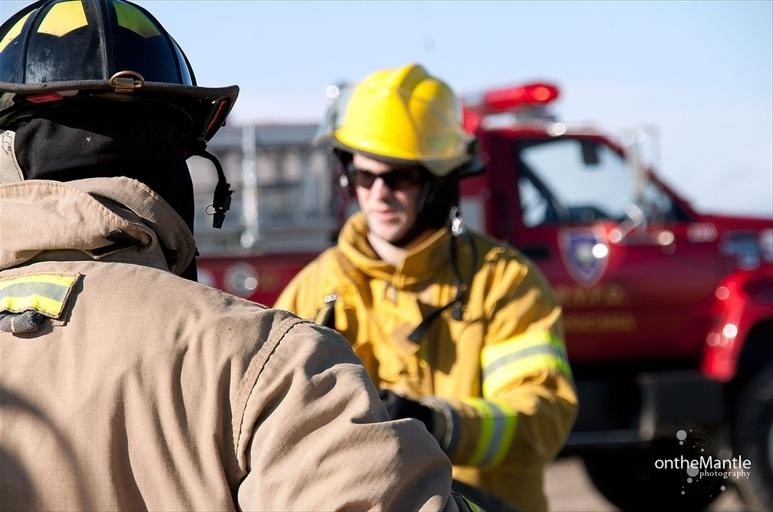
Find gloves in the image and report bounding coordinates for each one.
[378,388,437,440]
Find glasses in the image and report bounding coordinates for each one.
[347,163,424,191]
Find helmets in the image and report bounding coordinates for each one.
[0,0,240,141]
[330,63,475,177]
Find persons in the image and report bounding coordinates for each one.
[271,62,580,511]
[0,0,452,512]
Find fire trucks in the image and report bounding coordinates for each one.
[186,78,771,508]
